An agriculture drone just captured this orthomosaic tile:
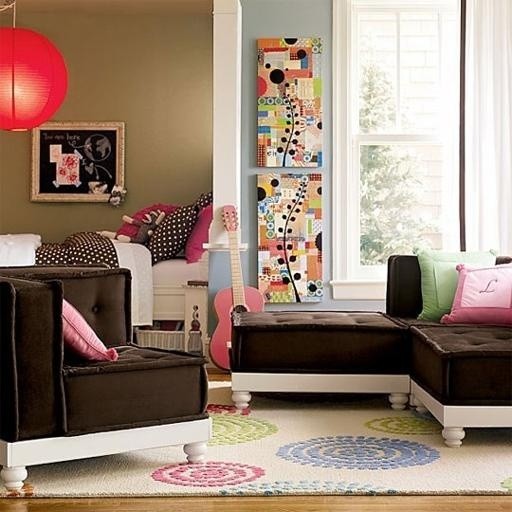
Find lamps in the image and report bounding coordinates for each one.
[0,0,67,131]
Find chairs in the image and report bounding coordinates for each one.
[0,262,213,491]
[386,255,512,408]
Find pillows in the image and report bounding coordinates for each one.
[115,191,213,265]
[61,298,119,363]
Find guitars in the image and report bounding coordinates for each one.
[209,206,264,370]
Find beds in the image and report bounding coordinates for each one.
[0,231,209,330]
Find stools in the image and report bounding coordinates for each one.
[228,309,410,411]
[409,325,512,448]
[413,246,497,321]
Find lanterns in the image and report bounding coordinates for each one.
[0,27,67,133]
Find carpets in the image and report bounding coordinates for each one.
[0,404,512,498]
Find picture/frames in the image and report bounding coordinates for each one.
[30,120,126,204]
